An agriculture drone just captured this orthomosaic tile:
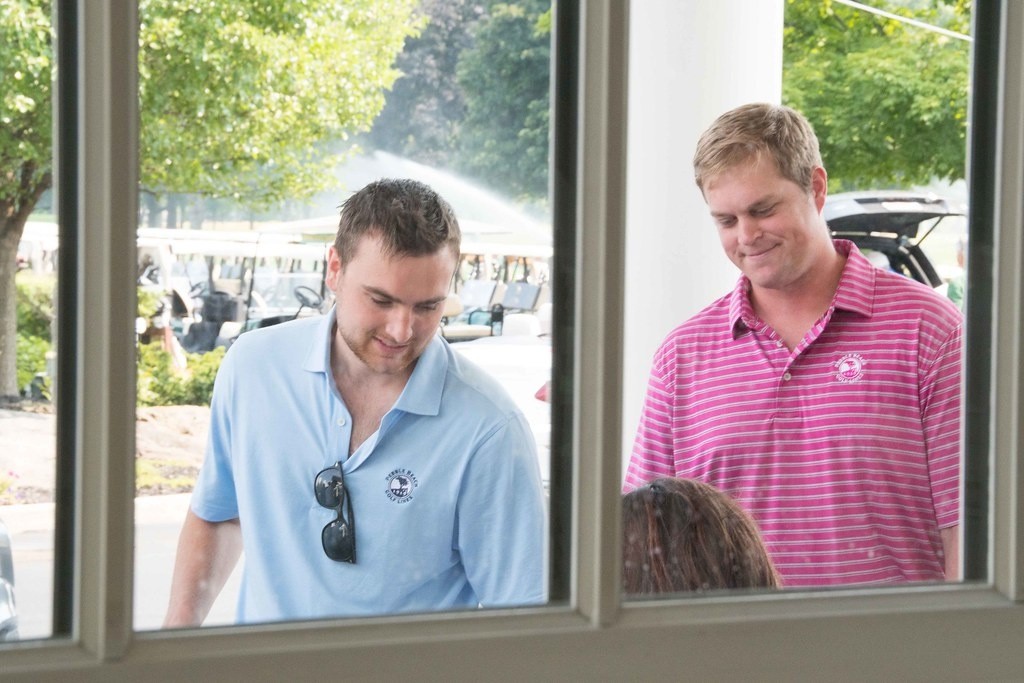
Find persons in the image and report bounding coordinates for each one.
[620,479,775,595]
[623,103,964,589]
[161,179,550,631]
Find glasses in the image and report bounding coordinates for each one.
[314,460,356,564]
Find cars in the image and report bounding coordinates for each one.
[531,191,965,425]
